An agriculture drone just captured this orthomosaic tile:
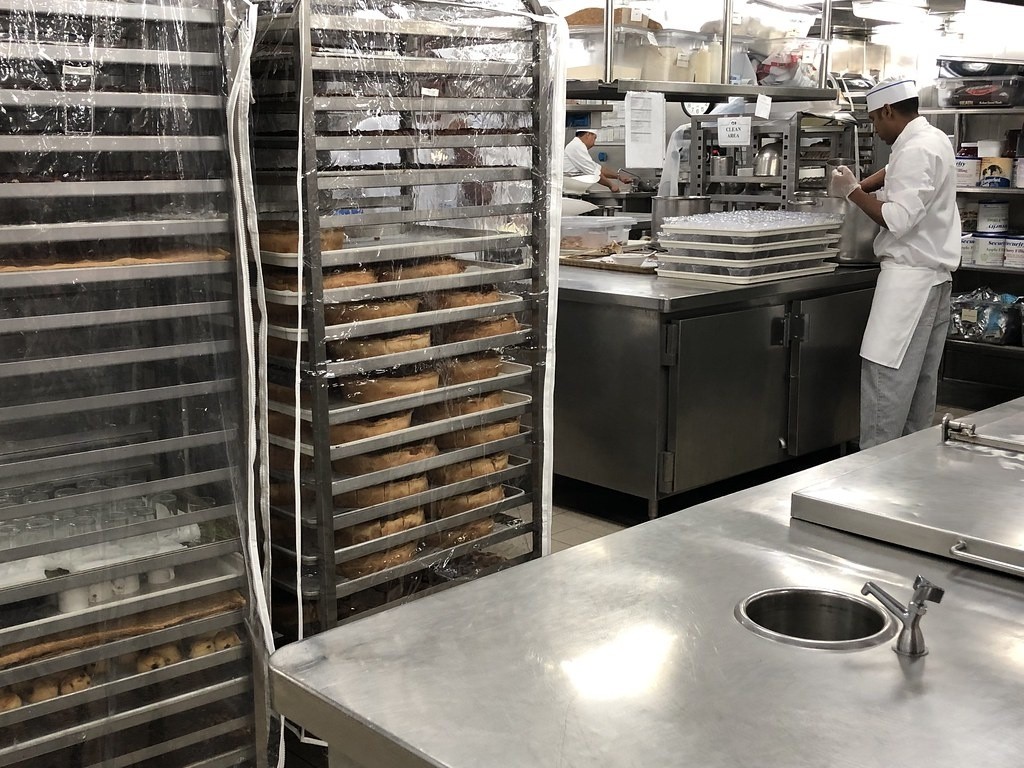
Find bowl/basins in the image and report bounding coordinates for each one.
[610,254,646,266]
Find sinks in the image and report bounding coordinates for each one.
[733,586,899,651]
[581,189,658,217]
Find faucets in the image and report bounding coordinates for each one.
[860,574,945,658]
[617,167,642,193]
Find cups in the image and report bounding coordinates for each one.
[710,155,735,175]
[826,158,857,199]
[0,472,239,613]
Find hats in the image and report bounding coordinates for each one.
[865,74,919,112]
[575,128,598,138]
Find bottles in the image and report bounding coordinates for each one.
[695,41,711,83]
[708,33,722,84]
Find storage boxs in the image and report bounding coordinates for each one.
[560,216,637,250]
[933,75,1024,108]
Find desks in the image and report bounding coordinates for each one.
[265,390,1024,768]
[553,263,881,521]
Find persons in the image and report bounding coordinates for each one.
[831,74,962,451]
[563,129,633,193]
[332,85,531,221]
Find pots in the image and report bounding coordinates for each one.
[787,189,882,266]
[650,195,711,249]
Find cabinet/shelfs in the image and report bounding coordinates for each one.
[0,1,549,768]
[918,109,1024,411]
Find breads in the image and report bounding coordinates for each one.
[258,229,521,582]
[0,567,243,716]
